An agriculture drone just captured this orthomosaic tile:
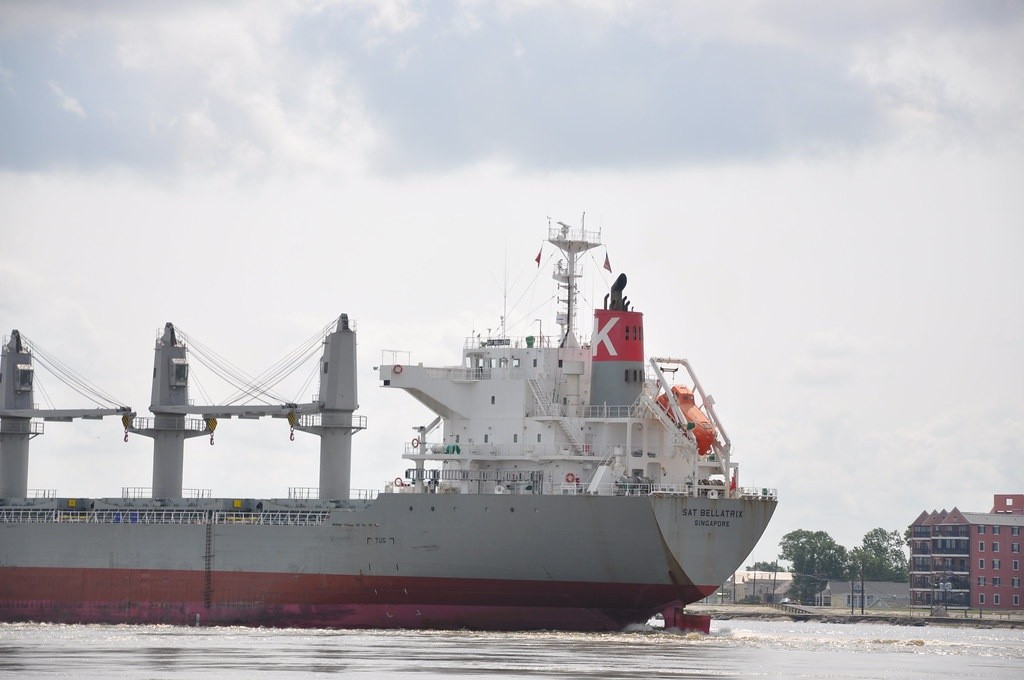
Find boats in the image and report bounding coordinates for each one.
[0,203,780,634]
[655,385,717,457]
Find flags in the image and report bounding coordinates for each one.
[602,250,613,273]
[534,247,542,269]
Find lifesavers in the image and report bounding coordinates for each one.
[412,439,418,447]
[395,478,402,486]
[566,473,575,482]
[394,364,403,372]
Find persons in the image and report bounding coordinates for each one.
[90,501,95,511]
